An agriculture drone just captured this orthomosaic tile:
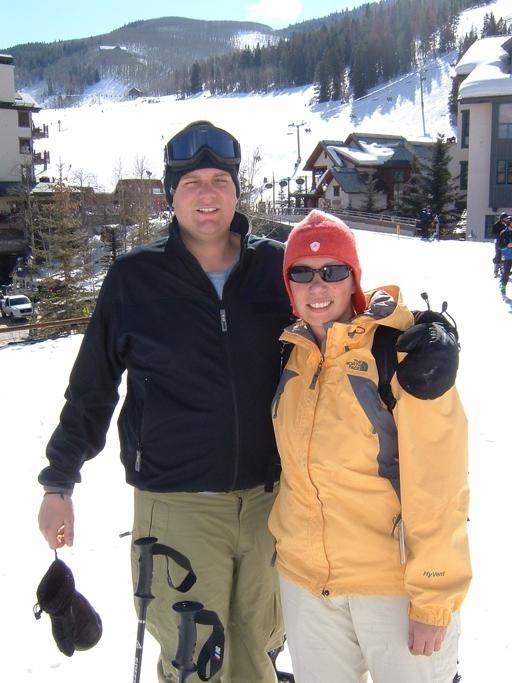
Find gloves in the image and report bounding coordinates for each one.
[36,560,102,656]
[396,293,460,400]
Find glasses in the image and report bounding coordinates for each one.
[166,124,239,170]
[287,264,353,282]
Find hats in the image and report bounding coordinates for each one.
[282,210,366,317]
[164,120,240,206]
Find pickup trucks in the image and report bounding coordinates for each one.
[1,295,37,324]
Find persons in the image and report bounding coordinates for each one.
[39,120,293,681]
[267,209,474,682]
[493,212,512,278]
[497,216,512,304]
[418,205,433,240]
